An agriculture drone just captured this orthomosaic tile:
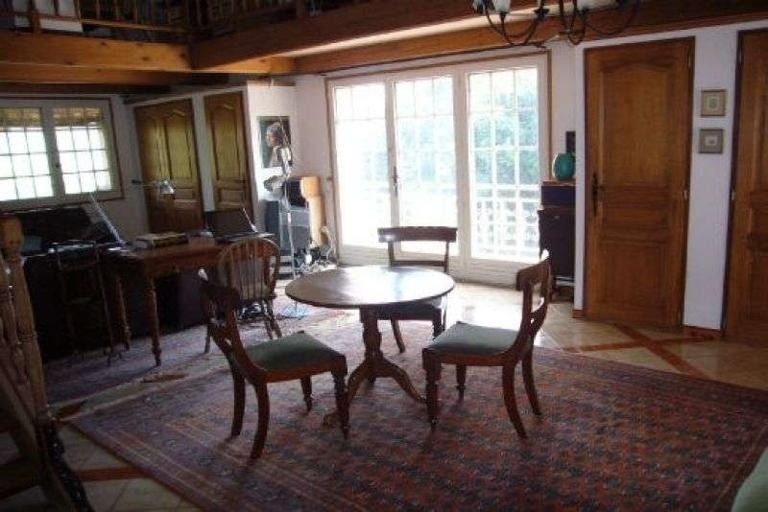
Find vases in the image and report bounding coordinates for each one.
[552,152,575,182]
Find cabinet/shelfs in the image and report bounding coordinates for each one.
[536,178,575,303]
[202,91,253,221]
[133,97,202,231]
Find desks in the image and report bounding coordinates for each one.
[100,228,276,366]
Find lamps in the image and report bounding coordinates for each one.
[156,179,175,197]
[469,0,638,50]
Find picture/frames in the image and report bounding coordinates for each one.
[698,128,723,153]
[700,89,727,116]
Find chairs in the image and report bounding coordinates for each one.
[48,239,122,367]
[377,226,458,352]
[198,281,351,458]
[422,249,551,439]
[199,238,281,355]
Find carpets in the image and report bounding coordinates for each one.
[42,295,768,511]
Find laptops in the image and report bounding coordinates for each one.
[204,205,276,240]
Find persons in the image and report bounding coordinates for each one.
[266,121,292,167]
